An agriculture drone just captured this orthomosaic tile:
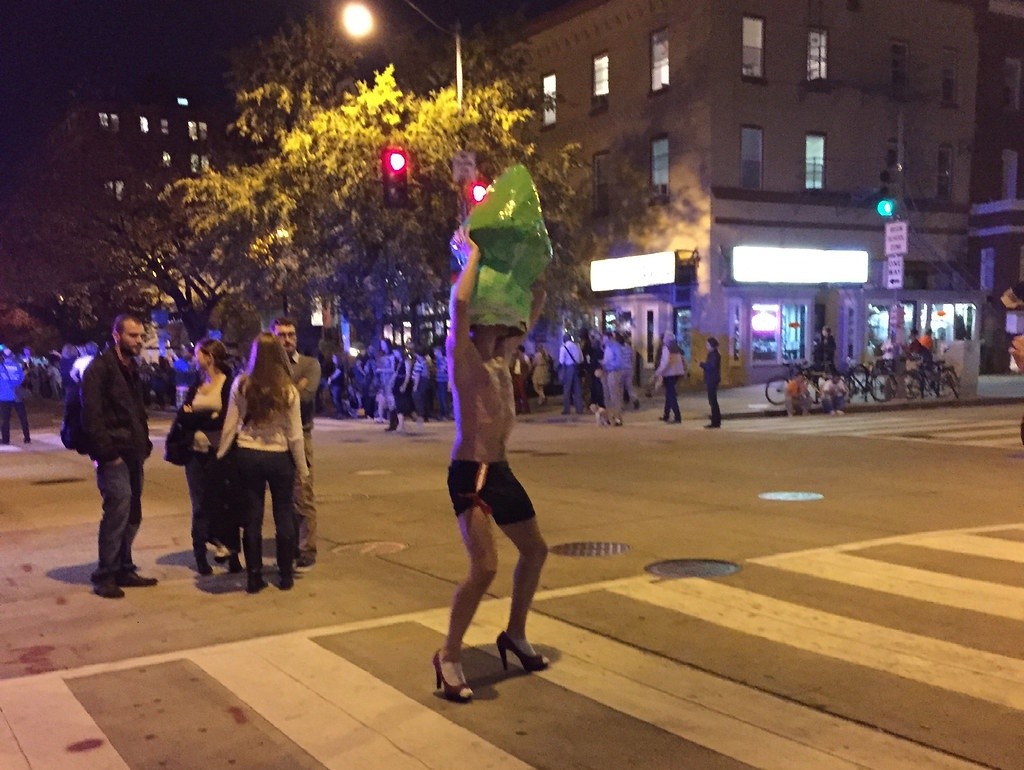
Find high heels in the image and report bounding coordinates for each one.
[432,646,473,703]
[496,631,549,673]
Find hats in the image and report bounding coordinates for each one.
[663,331,676,343]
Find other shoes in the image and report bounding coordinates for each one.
[830,409,845,417]
[293,548,316,567]
[24,438,31,444]
[197,552,243,577]
[0,438,10,445]
[659,414,682,424]
[704,422,721,429]
[245,573,293,594]
[93,571,158,598]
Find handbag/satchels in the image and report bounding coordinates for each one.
[575,361,590,379]
[387,370,397,392]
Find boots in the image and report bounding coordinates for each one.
[384,410,398,432]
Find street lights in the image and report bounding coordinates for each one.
[340,1,464,101]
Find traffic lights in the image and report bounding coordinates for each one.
[375,140,410,211]
[467,181,487,203]
[876,148,902,218]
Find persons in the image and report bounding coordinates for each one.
[510,328,684,426]
[217,332,308,591]
[697,337,722,428]
[786,325,933,421]
[432,225,549,702]
[323,337,453,430]
[180,337,243,575]
[269,317,322,567]
[1008,335,1024,372]
[79,313,157,598]
[0,342,243,445]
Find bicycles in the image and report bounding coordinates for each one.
[763,354,963,407]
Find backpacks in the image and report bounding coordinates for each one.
[57,360,122,451]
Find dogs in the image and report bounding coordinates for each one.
[589,403,621,426]
